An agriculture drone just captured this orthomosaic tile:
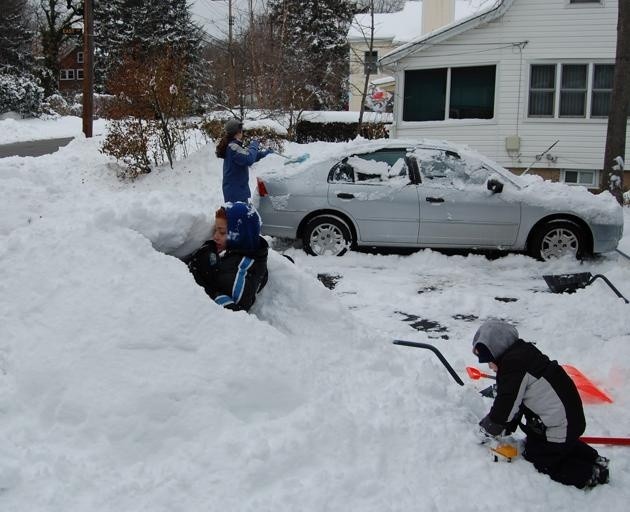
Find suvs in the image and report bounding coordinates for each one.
[365,76,396,115]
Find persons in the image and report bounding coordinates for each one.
[214,118,275,204]
[472,316,610,491]
[181,200,268,312]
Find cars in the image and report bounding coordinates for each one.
[253,141,626,265]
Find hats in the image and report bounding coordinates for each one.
[226,120,244,134]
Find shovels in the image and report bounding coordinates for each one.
[466,364,613,404]
